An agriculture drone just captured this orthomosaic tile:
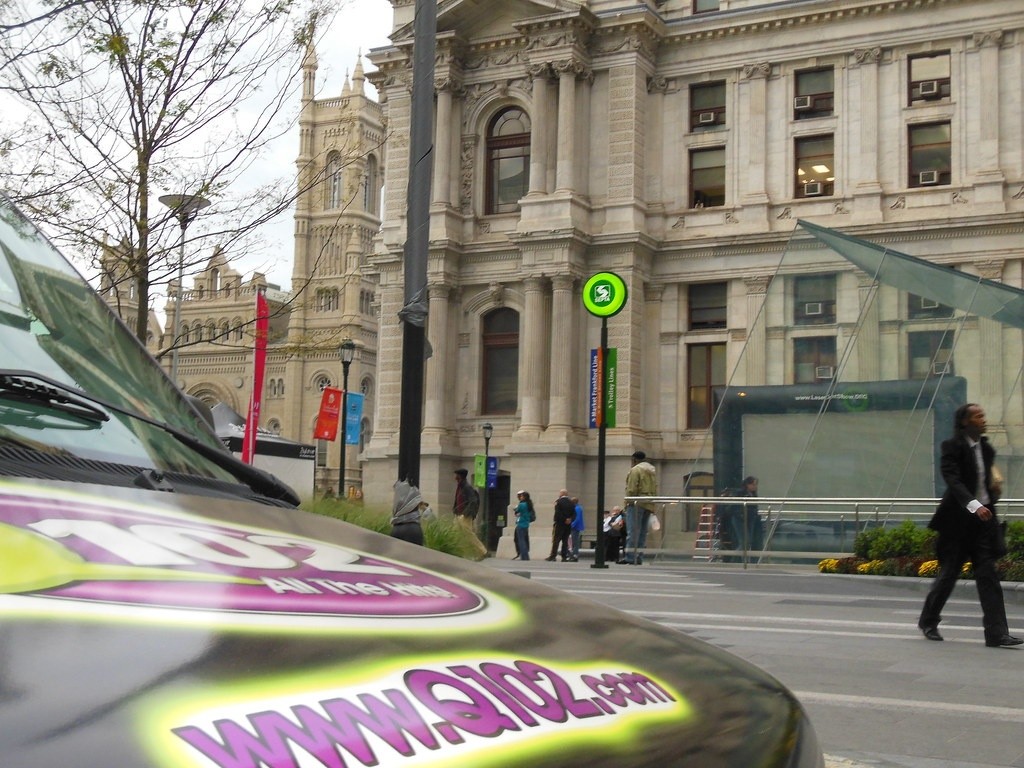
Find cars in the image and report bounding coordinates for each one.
[0,190,825,767]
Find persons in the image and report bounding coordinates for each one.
[615,450,657,565]
[917,403,1024,648]
[726,476,759,561]
[451,468,492,562]
[419,501,434,521]
[347,490,364,507]
[511,489,628,564]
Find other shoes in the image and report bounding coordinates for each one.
[545,555,556,561]
[618,559,633,564]
[562,555,568,562]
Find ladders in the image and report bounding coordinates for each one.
[692,504,714,562]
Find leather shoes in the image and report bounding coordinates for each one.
[986,635,1023,646]
[918,623,943,641]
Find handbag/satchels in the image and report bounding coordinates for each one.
[648,513,661,533]
[994,521,1009,561]
[531,510,535,522]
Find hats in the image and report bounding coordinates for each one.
[634,451,646,461]
[517,490,524,494]
[455,469,468,474]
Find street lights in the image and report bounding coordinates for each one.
[159,193,211,385]
[338,337,355,499]
[485,423,492,453]
